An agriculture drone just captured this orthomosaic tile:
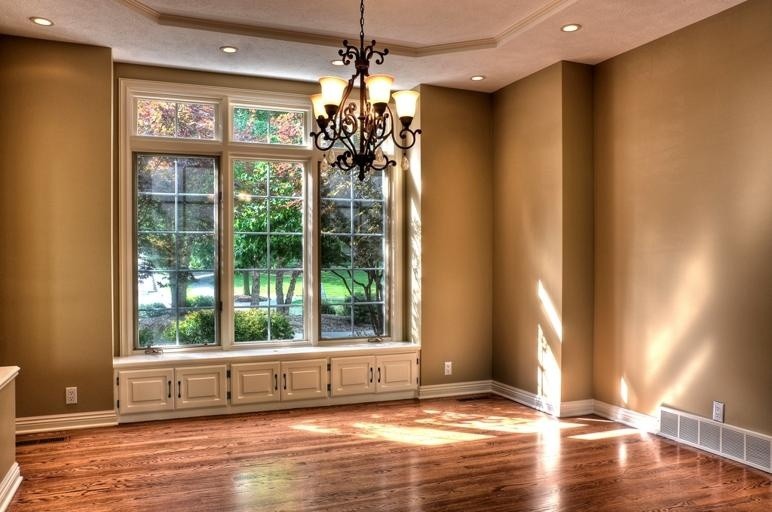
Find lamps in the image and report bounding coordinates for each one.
[306,0,422,180]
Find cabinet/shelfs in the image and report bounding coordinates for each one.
[329,353,417,403]
[229,356,329,414]
[117,358,229,422]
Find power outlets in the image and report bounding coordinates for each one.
[66,387,78,406]
[712,400,724,422]
